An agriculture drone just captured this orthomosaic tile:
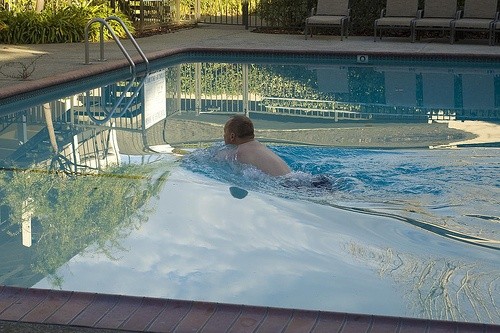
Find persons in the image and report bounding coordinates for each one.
[218,116,292,179]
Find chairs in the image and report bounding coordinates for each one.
[456,0,497,45]
[417,0,456,43]
[306,0,351,42]
[375,0,418,43]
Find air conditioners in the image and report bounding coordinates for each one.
[356,55,368,63]
